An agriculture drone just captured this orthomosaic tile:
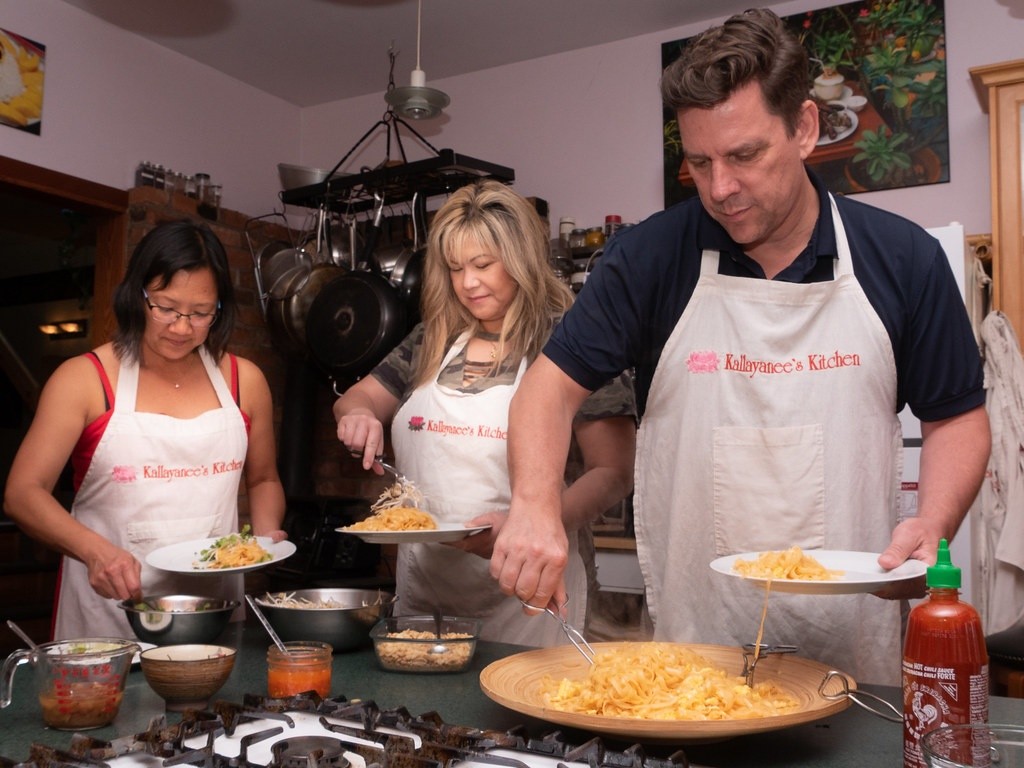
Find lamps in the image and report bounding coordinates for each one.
[382,0,451,123]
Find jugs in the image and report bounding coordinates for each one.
[1,621,142,732]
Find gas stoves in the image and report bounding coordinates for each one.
[0,691,706,768]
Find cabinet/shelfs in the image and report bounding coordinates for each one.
[963,52,1024,386]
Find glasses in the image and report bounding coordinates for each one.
[141,287,222,327]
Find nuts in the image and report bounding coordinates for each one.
[375,627,473,668]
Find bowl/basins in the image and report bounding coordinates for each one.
[826,100,845,117]
[255,587,398,653]
[919,723,1023,768]
[845,97,867,112]
[370,614,483,673]
[118,595,242,645]
[140,644,238,712]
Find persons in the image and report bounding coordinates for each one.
[335,180,638,648]
[488,10,992,687]
[6,220,288,638]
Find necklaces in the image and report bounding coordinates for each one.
[175,353,197,388]
[490,343,498,357]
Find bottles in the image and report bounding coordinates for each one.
[548,215,645,294]
[903,537,989,768]
[134,161,222,209]
[267,641,333,701]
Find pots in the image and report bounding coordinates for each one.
[252,195,435,375]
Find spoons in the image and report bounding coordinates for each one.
[427,611,449,655]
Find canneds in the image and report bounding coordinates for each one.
[604,215,622,243]
[560,217,575,249]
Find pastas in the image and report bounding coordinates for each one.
[535,641,803,724]
[348,505,438,531]
[208,541,263,568]
[733,545,832,658]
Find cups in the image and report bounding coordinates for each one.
[814,74,843,99]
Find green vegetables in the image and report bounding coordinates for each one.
[199,524,273,564]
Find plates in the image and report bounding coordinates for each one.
[709,550,930,596]
[479,641,855,741]
[337,524,492,543]
[809,87,853,99]
[815,109,858,146]
[47,641,158,665]
[148,537,296,577]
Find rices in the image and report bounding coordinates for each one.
[0,41,24,104]
